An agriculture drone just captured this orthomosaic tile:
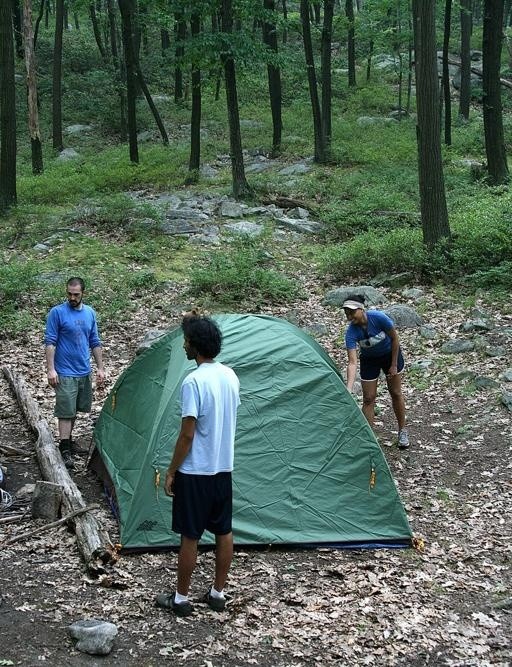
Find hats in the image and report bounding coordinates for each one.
[340,299,365,310]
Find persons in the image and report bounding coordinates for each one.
[43,277,105,468]
[156,314,241,617]
[339,295,409,449]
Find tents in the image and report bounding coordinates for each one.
[86,313,413,555]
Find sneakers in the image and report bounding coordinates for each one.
[70,441,88,455]
[203,589,226,611]
[61,450,74,468]
[155,591,191,616]
[398,430,409,447]
